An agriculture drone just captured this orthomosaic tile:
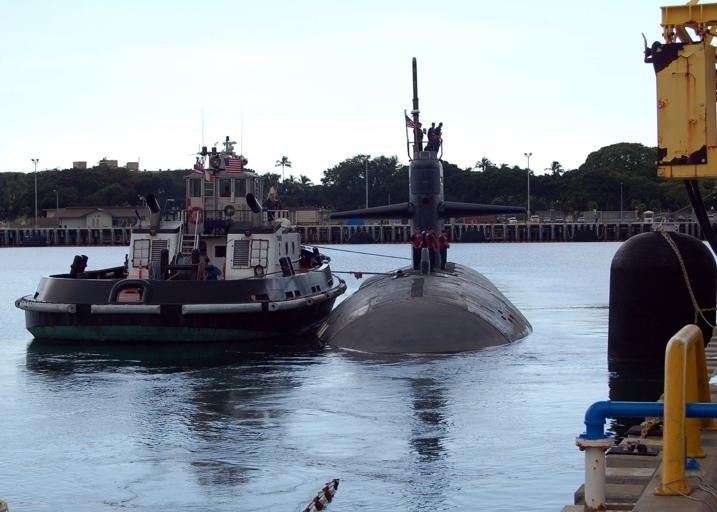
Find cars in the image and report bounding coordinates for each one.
[543,217,586,223]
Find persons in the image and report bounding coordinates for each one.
[413,123,443,151]
[267,184,278,201]
[410,227,449,272]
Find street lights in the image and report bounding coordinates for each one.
[360,155,371,208]
[53,188,59,225]
[524,152,533,220]
[31,159,39,227]
[620,181,623,220]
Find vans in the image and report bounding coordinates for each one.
[531,215,539,222]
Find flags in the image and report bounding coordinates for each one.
[406,115,416,128]
[224,158,241,173]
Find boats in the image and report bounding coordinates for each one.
[16,135,347,345]
